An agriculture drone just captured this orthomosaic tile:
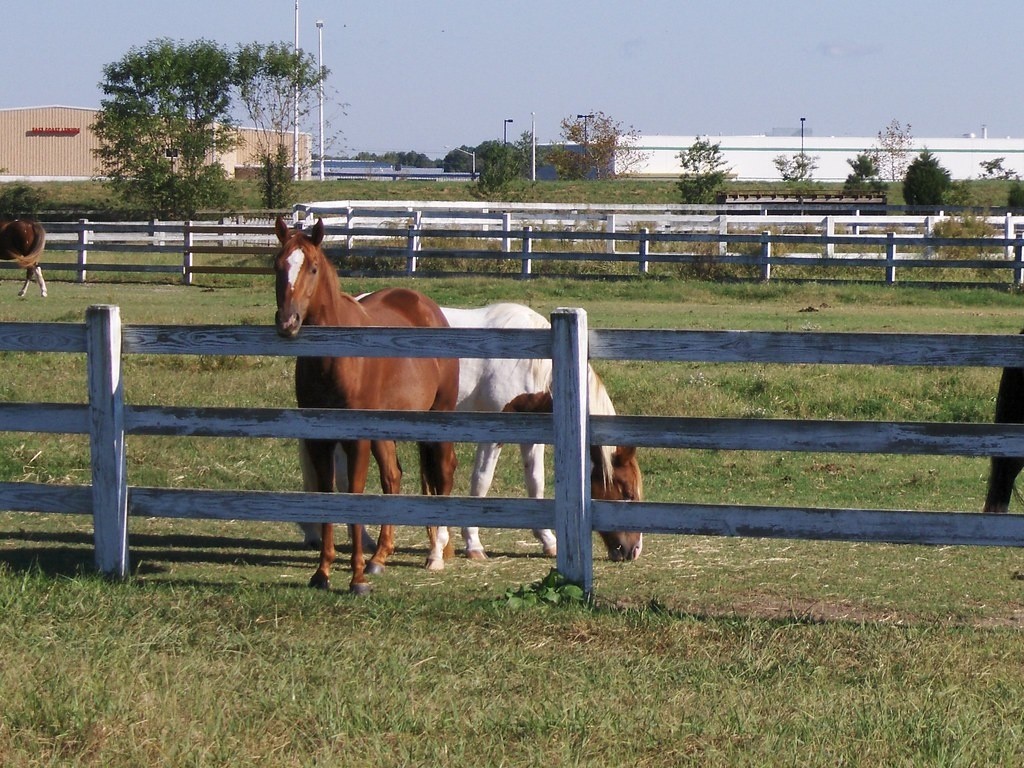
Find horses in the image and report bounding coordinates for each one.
[272,216,644,592]
[0,219,47,297]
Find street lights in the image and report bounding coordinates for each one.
[444,146,475,173]
[504,119,513,144]
[531,112,535,180]
[801,118,805,180]
[578,115,594,157]
[316,20,325,181]
